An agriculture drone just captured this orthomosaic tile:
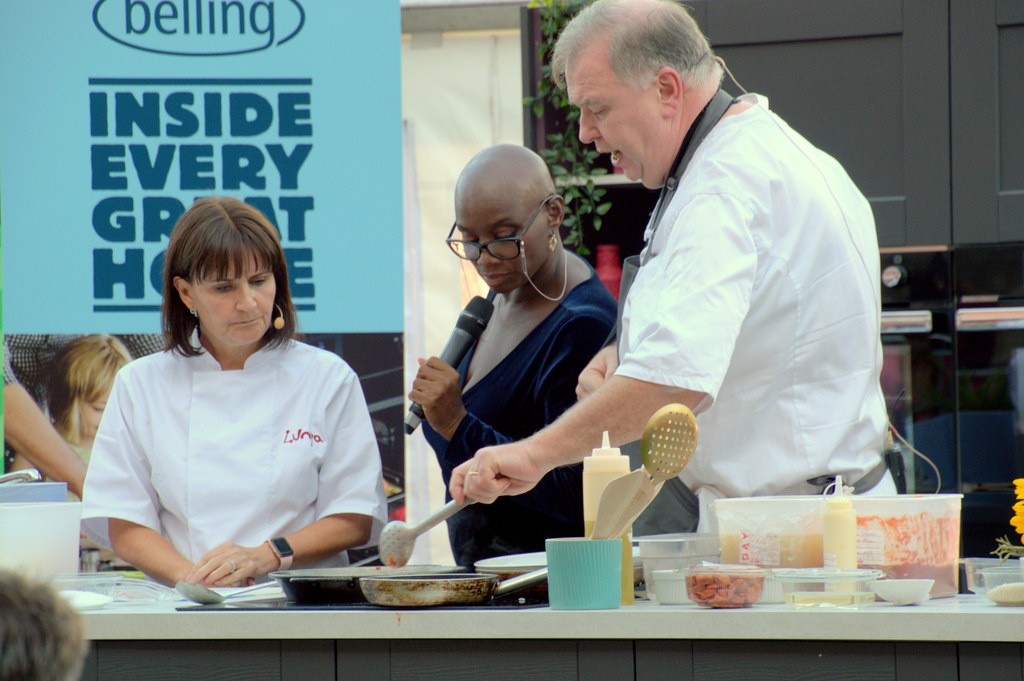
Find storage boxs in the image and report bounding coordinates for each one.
[0,482,83,589]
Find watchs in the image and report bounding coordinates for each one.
[263,536,293,571]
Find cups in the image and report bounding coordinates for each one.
[546,538,622,612]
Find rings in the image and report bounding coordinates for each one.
[467,471,478,474]
[227,559,236,573]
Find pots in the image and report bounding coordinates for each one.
[266,562,465,607]
[358,564,548,609]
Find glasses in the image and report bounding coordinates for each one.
[445,197,549,262]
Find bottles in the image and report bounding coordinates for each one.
[583,430,635,605]
[823,476,858,599]
[595,244,622,300]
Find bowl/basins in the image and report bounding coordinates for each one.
[774,568,884,610]
[628,531,718,606]
[960,558,1024,606]
[473,550,549,605]
[681,565,772,610]
[874,578,935,604]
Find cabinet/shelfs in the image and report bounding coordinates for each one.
[681,0,1024,247]
[520,0,660,302]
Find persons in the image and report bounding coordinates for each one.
[0,333,132,681]
[448,0,896,537]
[410,144,621,570]
[78,196,387,586]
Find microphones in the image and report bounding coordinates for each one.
[405,295,494,435]
